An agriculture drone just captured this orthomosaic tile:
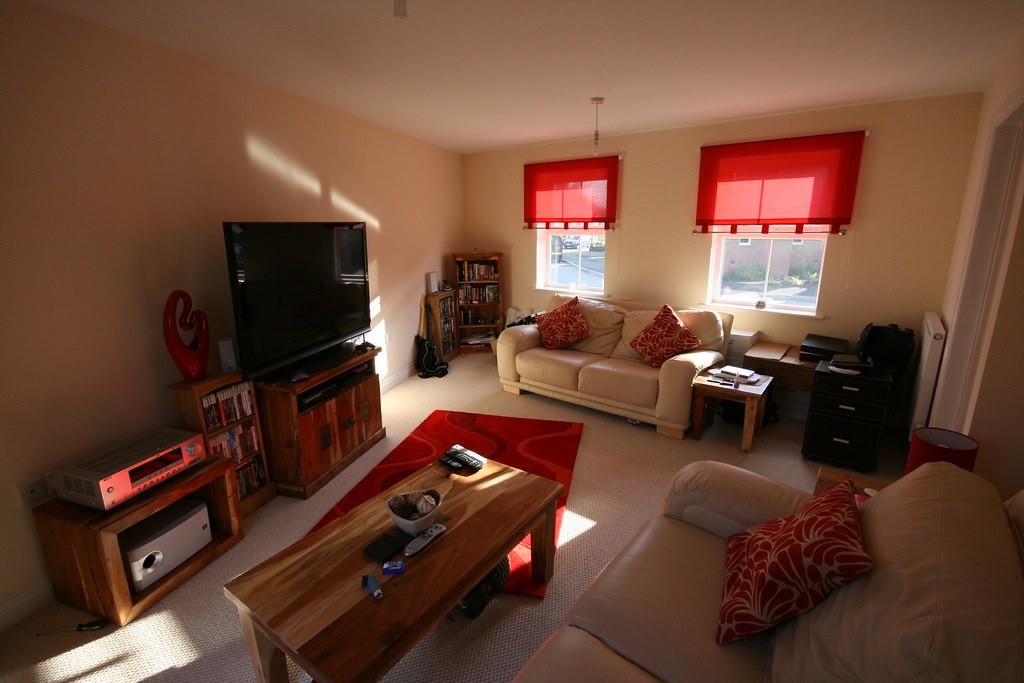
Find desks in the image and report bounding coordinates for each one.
[813,466,886,505]
[693,365,775,454]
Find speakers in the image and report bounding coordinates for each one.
[426,271,438,294]
[216,336,238,373]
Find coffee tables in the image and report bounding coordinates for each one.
[222,455,566,683]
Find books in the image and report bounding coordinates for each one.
[460,309,472,326]
[200,381,268,502]
[458,284,499,304]
[720,364,755,378]
[426,297,455,356]
[456,261,499,281]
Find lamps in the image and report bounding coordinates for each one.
[904,427,978,476]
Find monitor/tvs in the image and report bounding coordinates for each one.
[222,222,372,380]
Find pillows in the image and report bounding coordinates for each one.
[717,477,873,646]
[535,297,593,351]
[629,304,701,368]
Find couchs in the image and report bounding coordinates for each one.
[509,459,1024,683]
[495,292,734,439]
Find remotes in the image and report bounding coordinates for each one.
[404,523,447,556]
[439,444,488,470]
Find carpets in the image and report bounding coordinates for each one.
[307,410,583,600]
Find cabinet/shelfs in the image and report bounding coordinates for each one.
[800,360,892,474]
[426,251,504,362]
[32,456,245,627]
[254,348,387,499]
[165,370,277,518]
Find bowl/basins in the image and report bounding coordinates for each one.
[387,488,442,536]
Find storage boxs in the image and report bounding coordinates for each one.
[727,328,817,421]
